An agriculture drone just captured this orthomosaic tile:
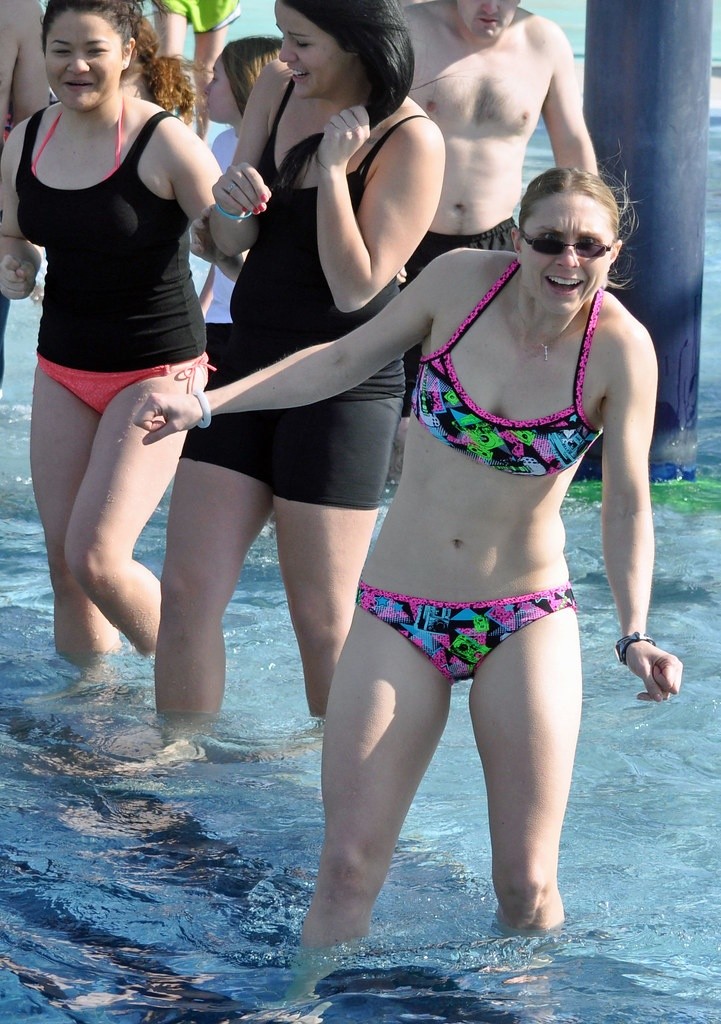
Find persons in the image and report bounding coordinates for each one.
[154,0,446,718]
[197,36,284,392]
[133,168,684,956]
[0,0,250,659]
[0,0,50,403]
[379,0,599,486]
[120,0,241,147]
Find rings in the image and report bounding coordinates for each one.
[228,182,236,194]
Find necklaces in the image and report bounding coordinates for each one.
[516,276,585,360]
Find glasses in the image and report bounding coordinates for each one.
[518,229,614,257]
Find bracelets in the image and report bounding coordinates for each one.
[214,203,253,221]
[615,632,656,666]
[193,389,211,428]
